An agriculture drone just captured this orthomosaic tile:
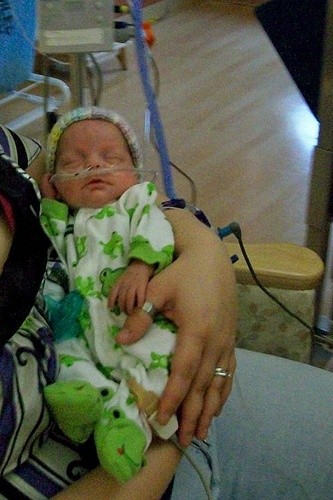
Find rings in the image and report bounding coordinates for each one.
[142,301,159,318]
[214,367,233,378]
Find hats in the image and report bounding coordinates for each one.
[42,106,143,181]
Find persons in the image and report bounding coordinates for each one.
[0,123,333,500]
[40,107,178,482]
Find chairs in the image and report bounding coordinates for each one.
[0,0,333,368]
